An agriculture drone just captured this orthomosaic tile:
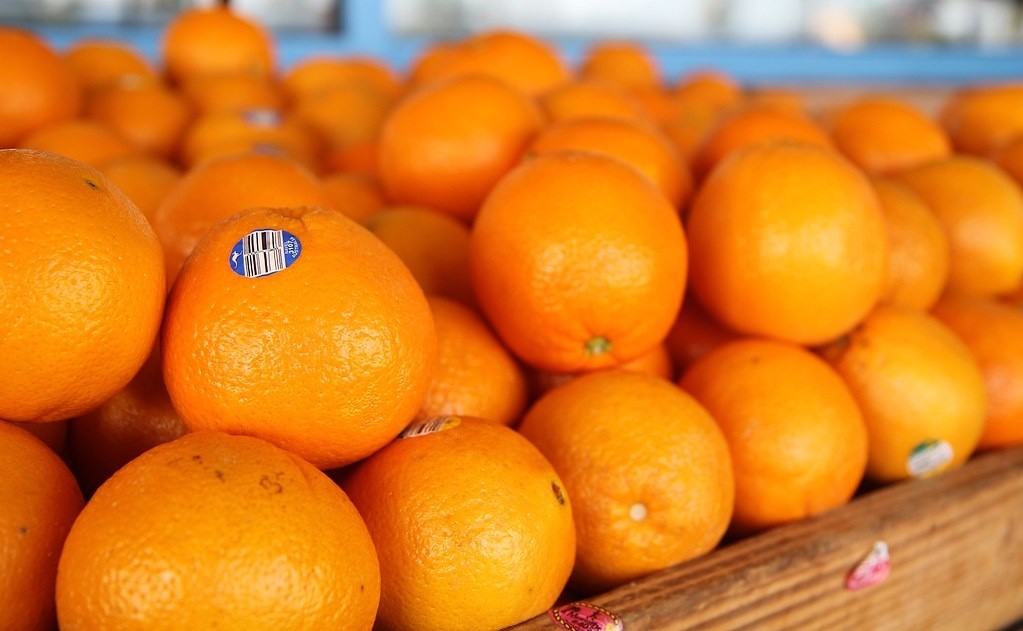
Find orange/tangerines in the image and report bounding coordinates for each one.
[6,0,1023,631]
